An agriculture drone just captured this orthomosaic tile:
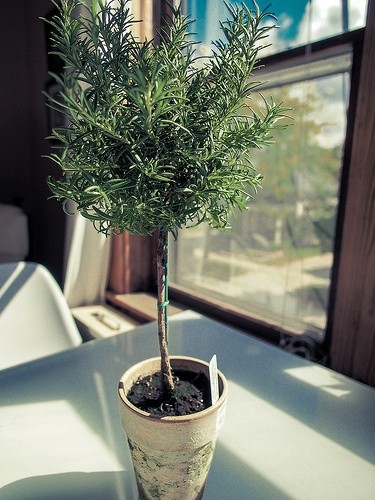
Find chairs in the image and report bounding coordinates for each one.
[0,261,83,371]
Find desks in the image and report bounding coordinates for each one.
[1,310,374,500]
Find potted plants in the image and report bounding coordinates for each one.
[39,0,294,500]
